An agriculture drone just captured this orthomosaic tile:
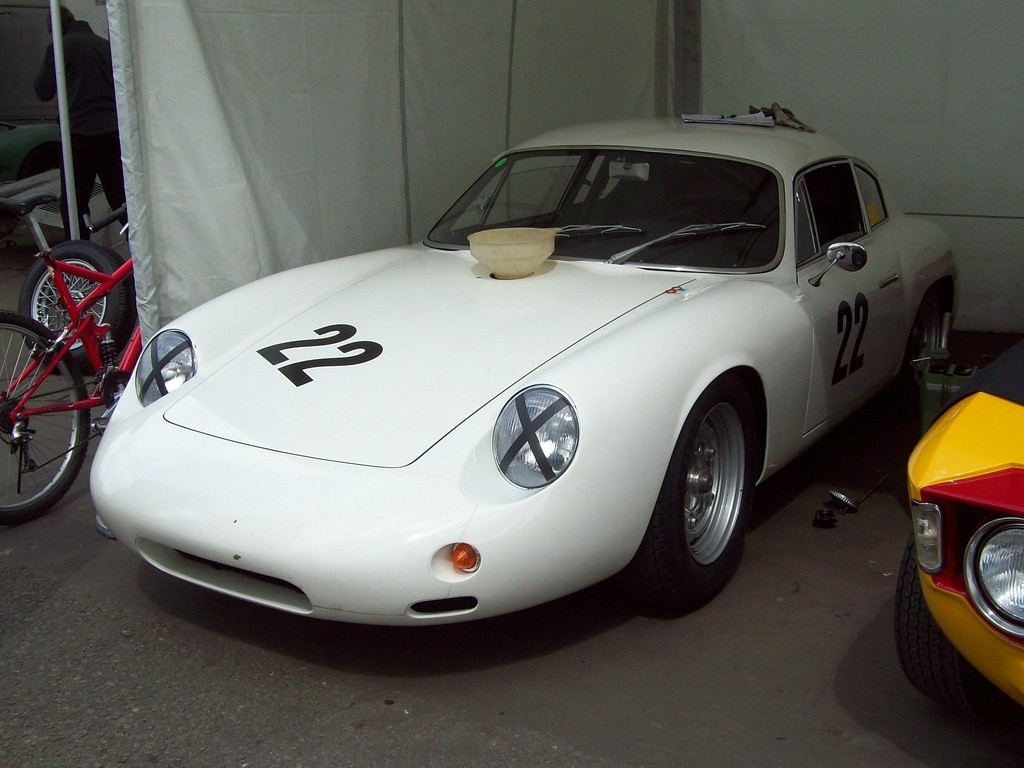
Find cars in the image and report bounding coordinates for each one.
[0,0,69,241]
[892,340,1024,714]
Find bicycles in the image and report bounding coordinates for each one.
[0,187,141,529]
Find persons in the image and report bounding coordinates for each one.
[36,6,128,242]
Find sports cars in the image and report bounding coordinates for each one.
[91,106,962,627]
